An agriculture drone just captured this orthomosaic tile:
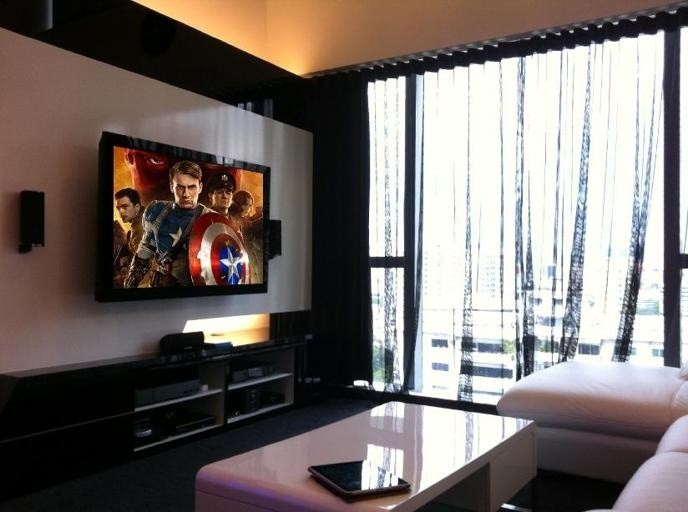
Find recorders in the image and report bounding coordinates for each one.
[160,332,204,357]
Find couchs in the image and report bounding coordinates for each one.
[496,361,688,512]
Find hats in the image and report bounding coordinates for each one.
[205,171,237,196]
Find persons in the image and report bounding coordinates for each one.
[115,148,255,288]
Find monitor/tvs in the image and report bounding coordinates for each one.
[95,132,270,302]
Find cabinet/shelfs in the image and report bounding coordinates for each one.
[0,341,328,503]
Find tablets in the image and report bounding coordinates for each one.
[308,460,410,499]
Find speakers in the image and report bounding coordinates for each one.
[271,310,328,408]
[18,191,45,252]
[269,220,281,257]
